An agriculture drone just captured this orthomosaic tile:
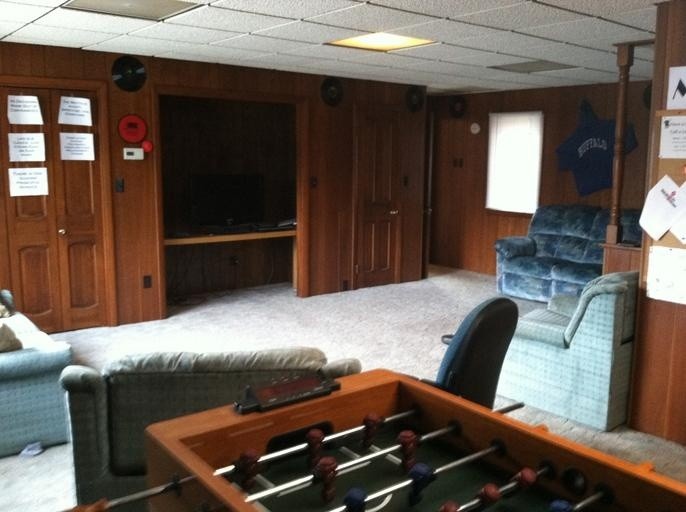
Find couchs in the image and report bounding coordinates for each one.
[54,345,364,512]
[487,199,646,307]
[1,286,76,455]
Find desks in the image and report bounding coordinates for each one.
[160,223,298,292]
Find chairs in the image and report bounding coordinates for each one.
[495,264,640,434]
[404,291,520,409]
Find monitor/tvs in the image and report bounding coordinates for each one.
[185,172,264,226]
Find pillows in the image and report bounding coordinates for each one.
[0,320,22,352]
[0,300,15,319]
